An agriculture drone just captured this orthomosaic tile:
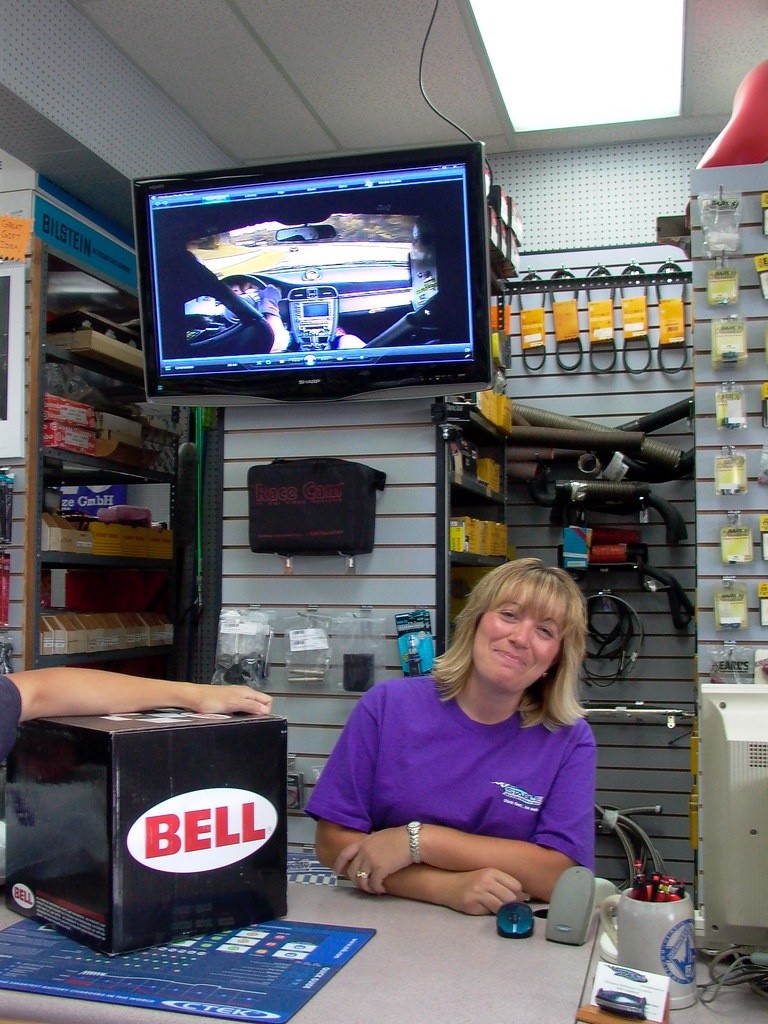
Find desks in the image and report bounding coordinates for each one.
[0,881,768,1024]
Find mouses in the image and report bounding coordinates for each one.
[496,901,534,939]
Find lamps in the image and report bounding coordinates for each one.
[695,60,768,170]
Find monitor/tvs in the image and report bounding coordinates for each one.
[131,141,494,407]
[700,683,768,965]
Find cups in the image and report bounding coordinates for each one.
[599,890,698,1010]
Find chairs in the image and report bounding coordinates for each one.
[182,250,275,359]
[363,293,440,349]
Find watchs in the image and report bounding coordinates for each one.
[406,821,422,864]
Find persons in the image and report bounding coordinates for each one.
[0,667,272,771]
[332,220,437,349]
[304,558,596,916]
[186,284,290,353]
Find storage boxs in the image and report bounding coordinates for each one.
[43,393,95,429]
[121,524,148,556]
[148,526,173,560]
[46,308,143,373]
[79,521,121,554]
[40,512,93,552]
[38,610,174,655]
[42,421,95,456]
[5,708,287,957]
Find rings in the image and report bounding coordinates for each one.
[355,870,369,878]
